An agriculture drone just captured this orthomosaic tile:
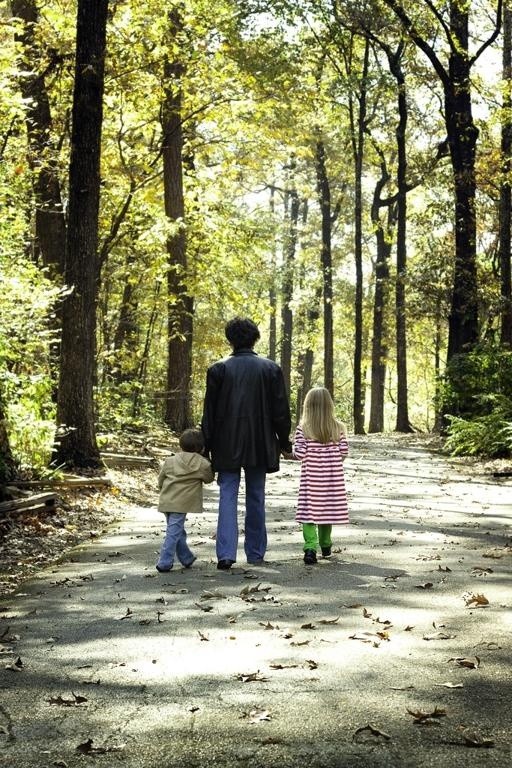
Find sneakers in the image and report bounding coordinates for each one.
[185,557,197,569]
[155,564,173,574]
[321,546,332,556]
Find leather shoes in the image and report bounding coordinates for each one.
[304,548,317,565]
[217,560,234,569]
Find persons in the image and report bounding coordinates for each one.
[155,427,214,572]
[200,315,293,569]
[282,385,350,563]
